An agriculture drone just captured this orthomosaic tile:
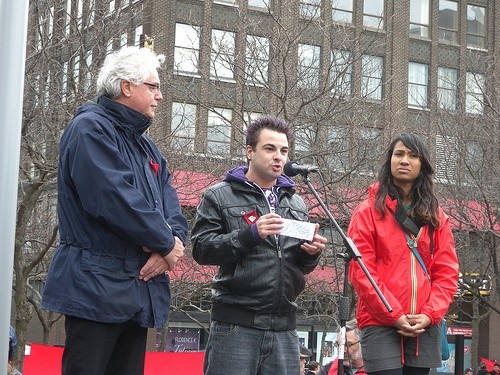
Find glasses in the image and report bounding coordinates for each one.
[334,341,360,349]
[142,81,160,92]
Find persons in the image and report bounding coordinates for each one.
[190,117,327,375]
[40,46,189,375]
[8,325,22,375]
[299,319,499,375]
[347,135,459,375]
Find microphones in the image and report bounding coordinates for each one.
[284,162,320,177]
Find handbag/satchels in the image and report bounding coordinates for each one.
[441,319,449,360]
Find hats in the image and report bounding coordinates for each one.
[300,344,312,358]
